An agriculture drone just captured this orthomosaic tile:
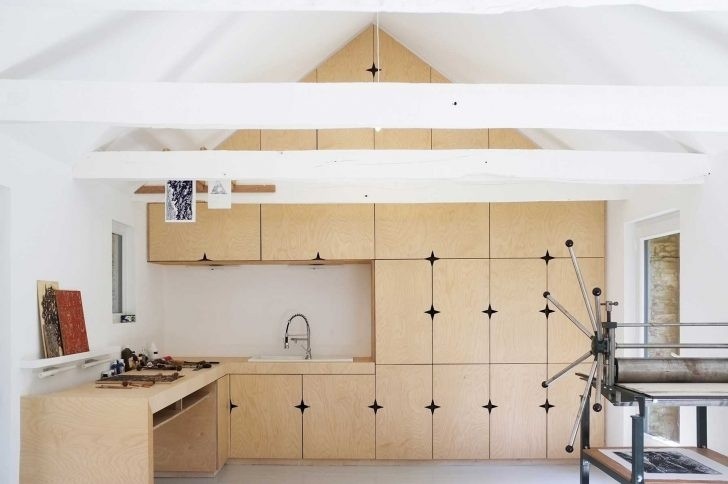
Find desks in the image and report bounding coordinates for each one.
[19,366,232,484]
[576,372,728,484]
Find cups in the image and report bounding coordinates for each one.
[175,359,184,372]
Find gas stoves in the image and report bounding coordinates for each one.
[94,373,185,389]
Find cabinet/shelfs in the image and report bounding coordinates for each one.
[146,26,610,462]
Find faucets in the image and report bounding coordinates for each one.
[283,313,312,359]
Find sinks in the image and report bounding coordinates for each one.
[248,355,354,362]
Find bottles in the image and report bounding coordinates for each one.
[132,351,139,368]
[121,346,134,371]
[152,352,160,360]
